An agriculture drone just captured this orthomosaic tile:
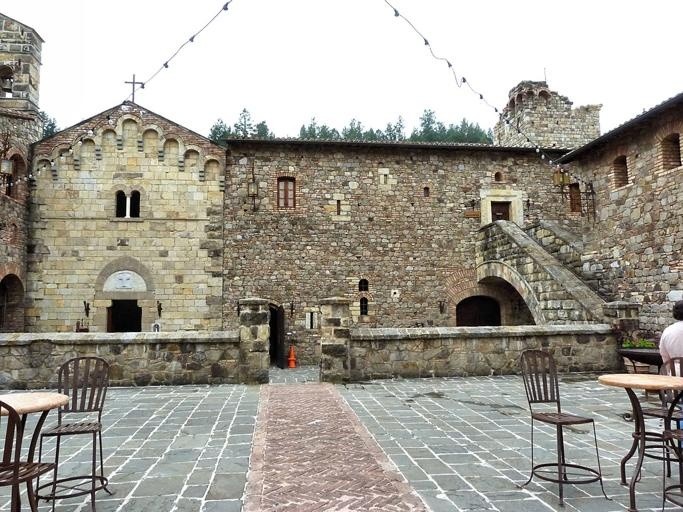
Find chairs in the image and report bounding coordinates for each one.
[33,357,117,512]
[642,356,682,478]
[662,428,683,511]
[515,349,612,505]
[0,401,55,511]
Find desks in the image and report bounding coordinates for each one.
[0,392,70,512]
[597,373,683,512]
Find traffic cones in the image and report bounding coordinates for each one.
[288,345,297,368]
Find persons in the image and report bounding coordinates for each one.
[659,299,683,449]
